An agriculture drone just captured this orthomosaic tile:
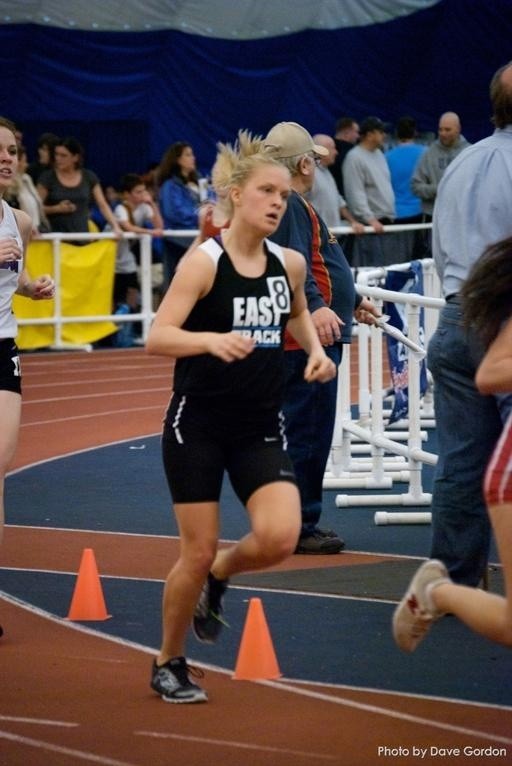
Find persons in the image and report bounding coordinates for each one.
[428,66,510,603]
[0,105,512,353]
[257,120,381,555]
[142,153,339,706]
[392,237,511,652]
[2,120,53,640]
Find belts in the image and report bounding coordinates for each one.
[447,296,473,305]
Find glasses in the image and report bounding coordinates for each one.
[297,156,321,166]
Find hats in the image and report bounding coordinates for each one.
[360,116,391,131]
[264,121,329,158]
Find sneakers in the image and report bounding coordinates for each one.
[191,571,228,643]
[392,559,449,653]
[295,527,344,554]
[151,657,208,703]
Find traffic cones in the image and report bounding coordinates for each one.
[229,597,287,680]
[61,546,112,622]
